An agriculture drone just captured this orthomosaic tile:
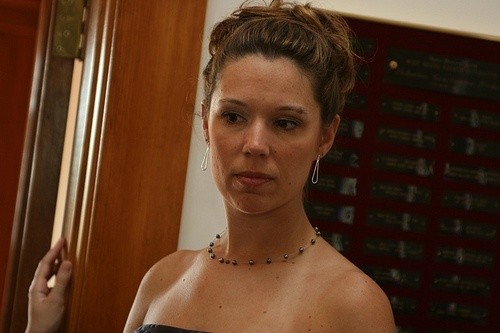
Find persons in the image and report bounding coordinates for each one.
[122,0,397,333]
[25,238,72,333]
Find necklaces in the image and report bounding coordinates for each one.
[208,234,320,265]
